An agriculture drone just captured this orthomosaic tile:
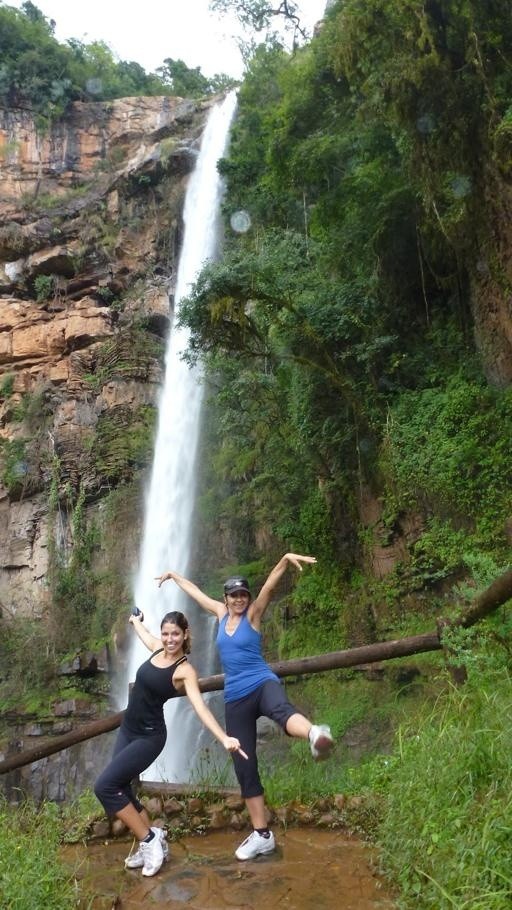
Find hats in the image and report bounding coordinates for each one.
[224,576,250,595]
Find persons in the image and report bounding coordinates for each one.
[155,553,335,860]
[94,611,250,877]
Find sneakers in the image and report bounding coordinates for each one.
[309,724,334,761]
[235,828,275,861]
[124,826,168,877]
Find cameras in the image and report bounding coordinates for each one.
[132,606,144,622]
